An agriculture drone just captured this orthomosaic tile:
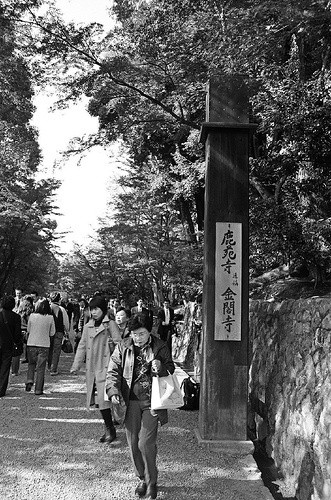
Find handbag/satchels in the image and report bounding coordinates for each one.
[109,340,114,356]
[150,370,184,409]
[12,342,24,357]
[62,337,73,353]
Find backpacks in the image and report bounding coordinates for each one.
[178,376,200,412]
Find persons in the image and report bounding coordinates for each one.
[69,296,132,443]
[105,312,175,499]
[0,285,176,397]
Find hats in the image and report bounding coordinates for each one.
[50,291,59,301]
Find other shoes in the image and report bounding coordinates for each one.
[35,392,44,395]
[99,426,116,443]
[22,359,28,364]
[12,371,21,376]
[48,369,59,375]
[25,382,33,392]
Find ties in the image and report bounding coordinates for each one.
[166,310,168,321]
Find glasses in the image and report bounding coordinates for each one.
[132,329,147,338]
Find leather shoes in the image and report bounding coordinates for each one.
[147,480,157,499]
[135,480,147,497]
